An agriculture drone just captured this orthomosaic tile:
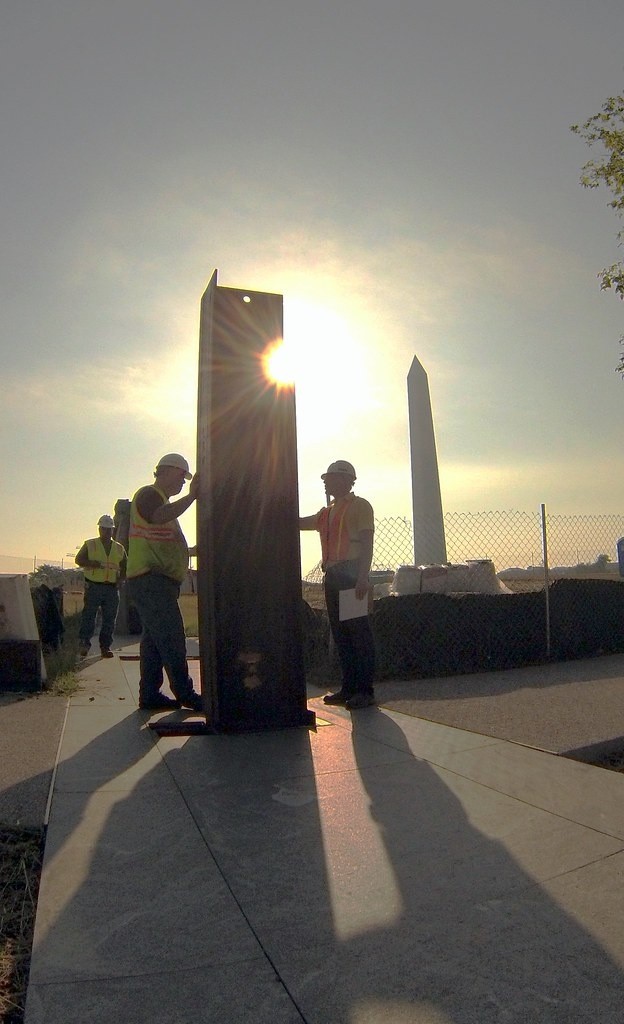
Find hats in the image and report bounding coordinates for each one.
[97,515,114,528]
[321,460,357,479]
[156,453,192,480]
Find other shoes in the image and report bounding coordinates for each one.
[102,649,114,658]
[176,694,202,708]
[139,695,181,709]
[80,648,88,656]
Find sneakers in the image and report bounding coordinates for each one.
[346,696,376,708]
[324,694,350,704]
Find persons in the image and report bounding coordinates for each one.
[299,459,377,709]
[76,514,127,658]
[125,453,204,711]
